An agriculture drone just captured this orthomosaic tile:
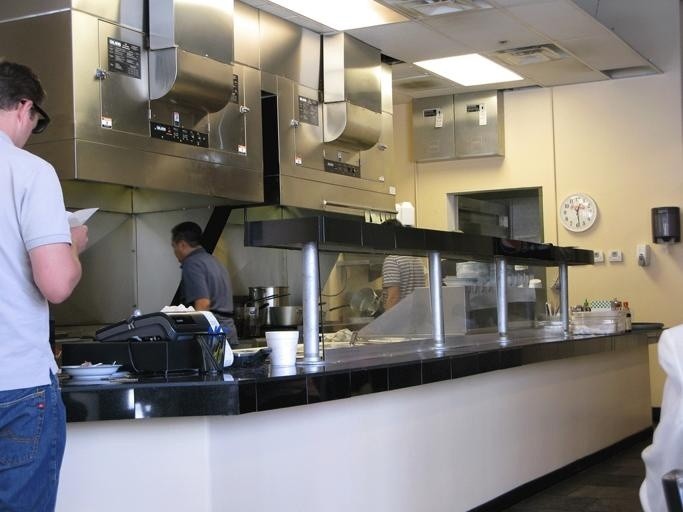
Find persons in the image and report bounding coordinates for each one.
[0,58,89,511]
[168,219,240,353]
[372,218,426,312]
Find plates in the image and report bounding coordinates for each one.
[59,362,123,380]
[442,260,494,286]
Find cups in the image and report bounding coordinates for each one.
[195,333,226,378]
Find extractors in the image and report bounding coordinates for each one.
[258,6,398,226]
[0,0,264,216]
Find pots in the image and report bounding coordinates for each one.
[248,286,290,326]
[233,302,270,320]
[317,303,349,324]
[269,302,327,327]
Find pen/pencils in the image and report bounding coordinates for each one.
[545,302,560,317]
[195,324,231,373]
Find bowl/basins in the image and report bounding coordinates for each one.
[350,287,380,317]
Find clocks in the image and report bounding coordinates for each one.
[558,193,597,233]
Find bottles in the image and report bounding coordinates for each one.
[130,303,142,317]
[622,302,633,331]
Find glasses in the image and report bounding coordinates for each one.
[20,100,50,134]
